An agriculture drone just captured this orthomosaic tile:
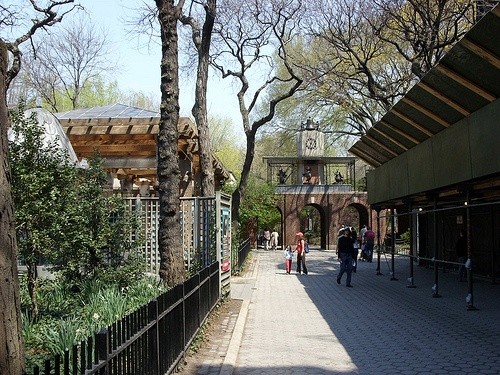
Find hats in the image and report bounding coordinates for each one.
[297,232,303,236]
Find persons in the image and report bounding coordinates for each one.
[333,169,344,183]
[363,226,375,263]
[276,168,286,185]
[263,227,271,251]
[337,227,354,288]
[271,228,278,252]
[284,245,294,274]
[303,165,312,184]
[293,232,307,276]
[336,225,358,272]
[360,225,367,242]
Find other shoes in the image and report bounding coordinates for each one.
[302,273,307,275]
[346,284,353,288]
[337,276,340,284]
[287,272,290,275]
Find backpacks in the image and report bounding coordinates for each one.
[300,238,309,253]
